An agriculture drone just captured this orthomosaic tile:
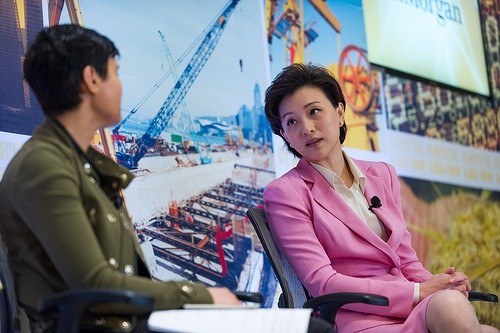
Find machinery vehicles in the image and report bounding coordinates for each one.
[105,0,245,180]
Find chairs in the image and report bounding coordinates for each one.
[0,245,263,333]
[247,207,499,333]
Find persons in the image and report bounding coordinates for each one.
[0,25,242,333]
[263,62,500,333]
